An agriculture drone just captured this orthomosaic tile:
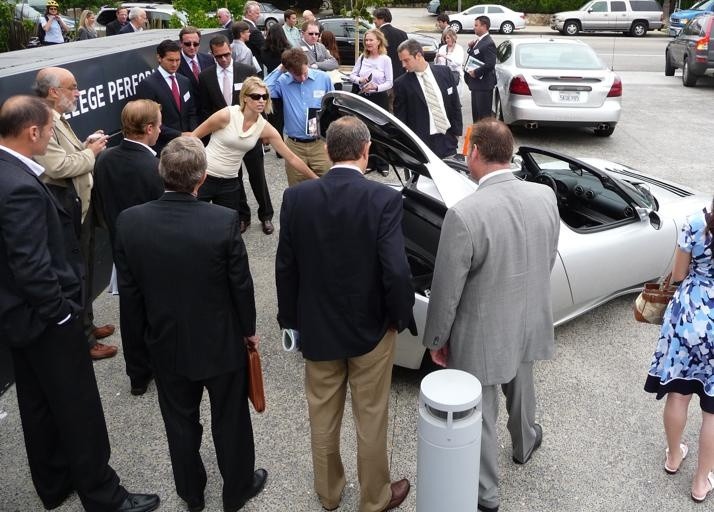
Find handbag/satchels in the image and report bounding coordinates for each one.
[632,271,681,324]
[242,342,266,413]
[351,54,364,93]
[446,46,460,84]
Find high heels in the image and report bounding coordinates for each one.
[366,168,375,174]
[383,170,389,178]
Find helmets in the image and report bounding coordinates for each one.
[45,1,59,6]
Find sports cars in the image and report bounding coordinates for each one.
[316,90,712,376]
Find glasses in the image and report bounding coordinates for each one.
[183,41,200,47]
[305,32,319,35]
[212,48,232,59]
[244,92,269,101]
[48,8,59,11]
[216,15,227,19]
[57,85,79,93]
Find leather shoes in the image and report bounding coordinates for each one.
[111,493,160,512]
[239,220,251,232]
[478,503,500,512]
[187,493,205,511]
[89,341,119,359]
[262,220,274,234]
[514,424,542,463]
[387,479,409,509]
[93,324,115,339]
[223,468,267,512]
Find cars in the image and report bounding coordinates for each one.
[427,0,442,15]
[437,5,526,34]
[204,2,286,30]
[664,13,714,86]
[491,36,622,137]
[316,17,440,65]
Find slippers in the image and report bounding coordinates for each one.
[665,443,689,472]
[691,469,714,500]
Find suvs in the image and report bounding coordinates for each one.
[90,3,192,37]
[550,0,665,37]
[14,2,80,49]
[668,1,714,37]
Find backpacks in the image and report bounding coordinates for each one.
[36,15,50,42]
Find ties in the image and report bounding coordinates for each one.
[192,61,200,83]
[422,72,448,135]
[222,70,232,106]
[60,115,77,138]
[170,76,181,111]
[464,39,477,66]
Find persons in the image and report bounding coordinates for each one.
[0,96,161,512]
[423,117,561,510]
[114,136,267,512]
[186,77,321,224]
[264,48,336,186]
[75,9,96,41]
[229,22,254,67]
[434,29,465,87]
[645,194,714,489]
[177,28,219,129]
[216,8,235,36]
[29,67,119,359]
[466,15,498,124]
[295,21,338,74]
[119,8,148,37]
[137,39,199,140]
[275,115,417,512]
[318,31,339,60]
[260,26,288,158]
[95,99,168,395]
[348,28,393,177]
[39,0,68,44]
[243,2,260,35]
[282,10,300,46]
[372,7,408,76]
[393,39,463,158]
[200,36,274,234]
[302,10,317,23]
[106,9,128,38]
[437,13,457,42]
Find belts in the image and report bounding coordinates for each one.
[289,137,322,143]
